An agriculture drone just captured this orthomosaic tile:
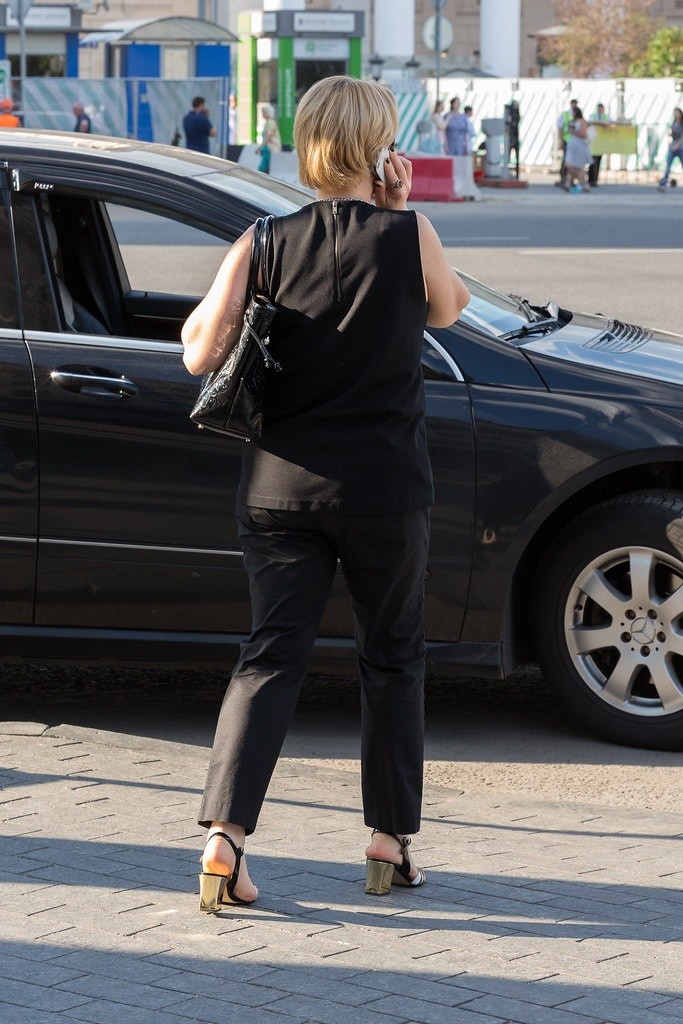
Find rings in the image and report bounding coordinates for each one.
[389,179,403,191]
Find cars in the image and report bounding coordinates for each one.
[1,126,683,757]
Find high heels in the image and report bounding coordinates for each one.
[198,832,259,912]
[364,828,426,895]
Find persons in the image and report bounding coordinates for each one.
[657,107,683,191]
[182,97,217,156]
[0,97,21,128]
[555,100,608,196]
[73,102,91,134]
[228,94,236,146]
[505,100,521,181]
[428,98,475,156]
[182,76,470,919]
[255,104,283,173]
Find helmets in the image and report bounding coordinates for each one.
[0,98,13,108]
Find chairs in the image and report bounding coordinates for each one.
[42,211,110,336]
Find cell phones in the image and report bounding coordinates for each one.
[372,146,392,186]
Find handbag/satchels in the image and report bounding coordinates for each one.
[188,214,282,442]
